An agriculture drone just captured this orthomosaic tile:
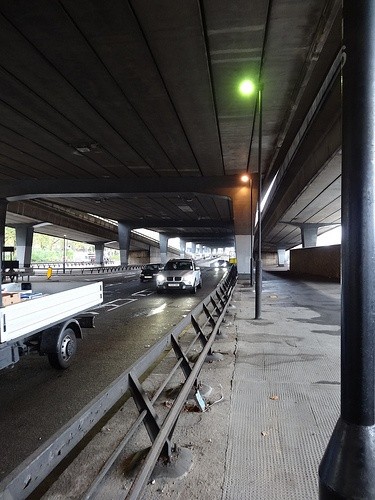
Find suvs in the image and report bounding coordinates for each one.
[152,258,203,294]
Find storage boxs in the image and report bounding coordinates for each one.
[0,291,21,307]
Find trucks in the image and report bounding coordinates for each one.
[0,279,104,371]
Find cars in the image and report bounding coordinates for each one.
[140,263,165,282]
[215,260,226,267]
